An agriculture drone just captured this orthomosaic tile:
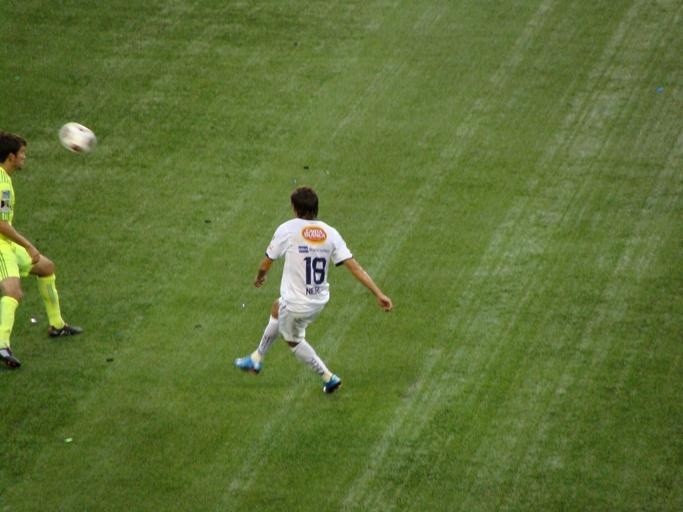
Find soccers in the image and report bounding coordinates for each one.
[59,122,96,155]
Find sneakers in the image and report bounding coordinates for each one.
[47,323,83,337]
[0,348,20,369]
[320,374,342,395]
[234,355,261,374]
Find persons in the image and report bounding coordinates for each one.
[235,187,393,395]
[0,133,83,369]
